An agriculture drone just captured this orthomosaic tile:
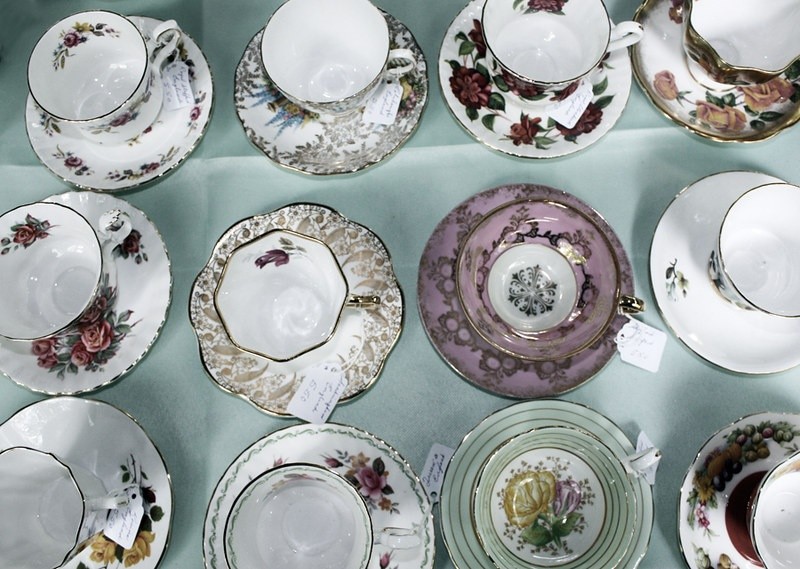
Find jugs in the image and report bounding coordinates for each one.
[0,202,132,344]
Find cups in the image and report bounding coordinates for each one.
[223,463,421,569]
[1,446,131,568]
[481,0,644,92]
[26,9,184,145]
[454,195,645,361]
[470,425,638,569]
[745,448,800,568]
[212,227,381,364]
[682,0,799,94]
[260,0,415,117]
[709,183,799,320]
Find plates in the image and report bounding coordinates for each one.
[649,170,799,376]
[440,398,655,569]
[0,395,174,568]
[438,1,633,161]
[629,0,800,144]
[416,185,634,399]
[24,16,214,192]
[189,201,406,419]
[201,422,435,569]
[1,192,174,394]
[233,7,429,177]
[677,411,799,567]
